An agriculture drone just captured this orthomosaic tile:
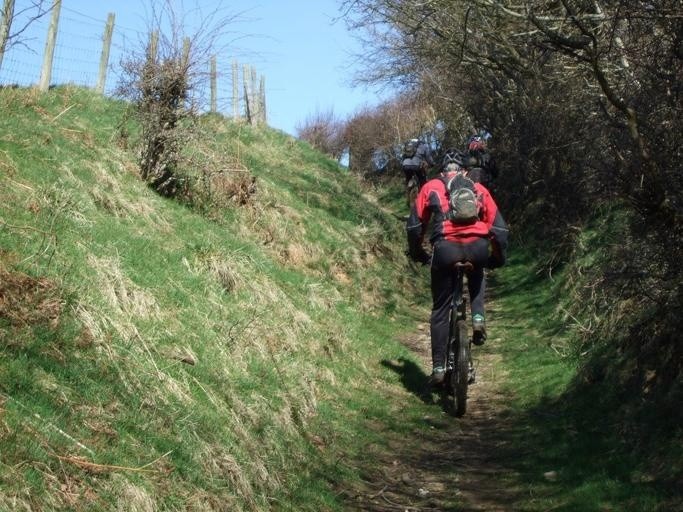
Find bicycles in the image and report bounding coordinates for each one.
[406,174,418,209]
[428,261,485,417]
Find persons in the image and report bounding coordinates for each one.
[405,151,508,387]
[399,118,493,206]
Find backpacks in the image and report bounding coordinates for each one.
[401,138,421,159]
[434,172,482,226]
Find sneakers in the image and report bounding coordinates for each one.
[424,368,447,389]
[471,320,488,346]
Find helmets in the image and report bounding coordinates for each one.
[436,146,469,173]
